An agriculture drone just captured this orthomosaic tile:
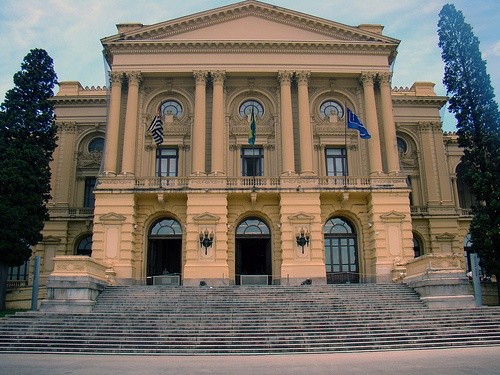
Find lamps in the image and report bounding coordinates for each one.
[199,227,214,255]
[295,226,310,254]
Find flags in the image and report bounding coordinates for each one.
[346,107,371,139]
[147,115,164,146]
[247,105,256,145]
